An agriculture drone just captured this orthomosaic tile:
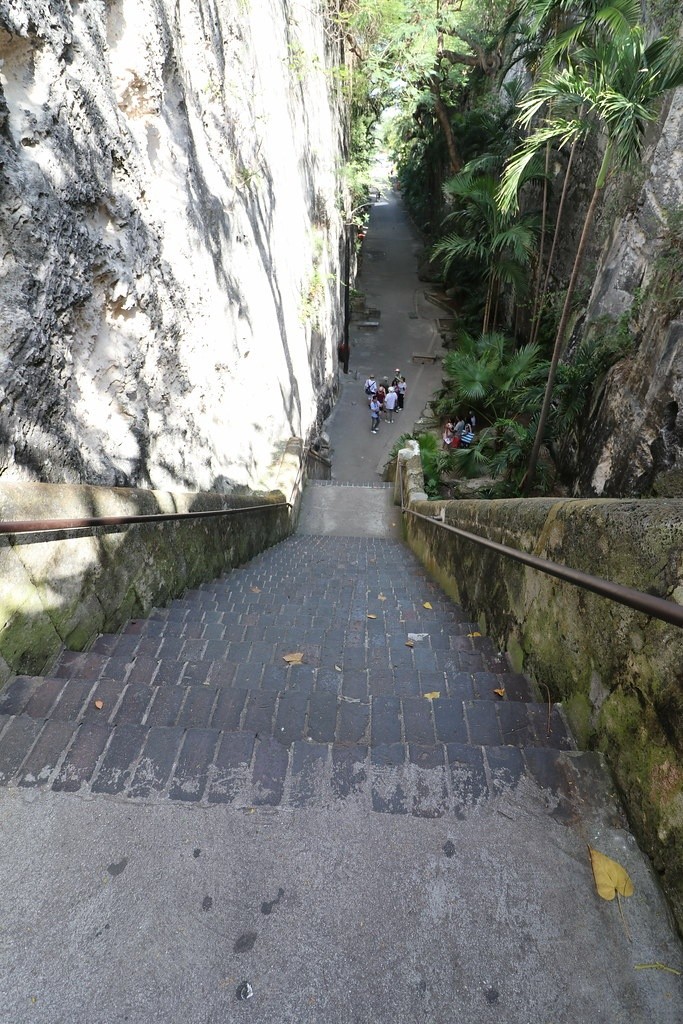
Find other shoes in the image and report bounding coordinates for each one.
[395,406,403,413]
[379,410,382,414]
[385,418,394,424]
[371,428,380,434]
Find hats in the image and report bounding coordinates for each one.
[388,386,394,392]
[382,375,389,381]
[394,368,401,373]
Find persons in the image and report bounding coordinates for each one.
[357,225,364,234]
[364,369,407,434]
[443,411,475,448]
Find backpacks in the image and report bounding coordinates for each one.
[365,380,376,395]
[376,388,385,400]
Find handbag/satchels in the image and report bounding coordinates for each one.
[460,425,475,443]
